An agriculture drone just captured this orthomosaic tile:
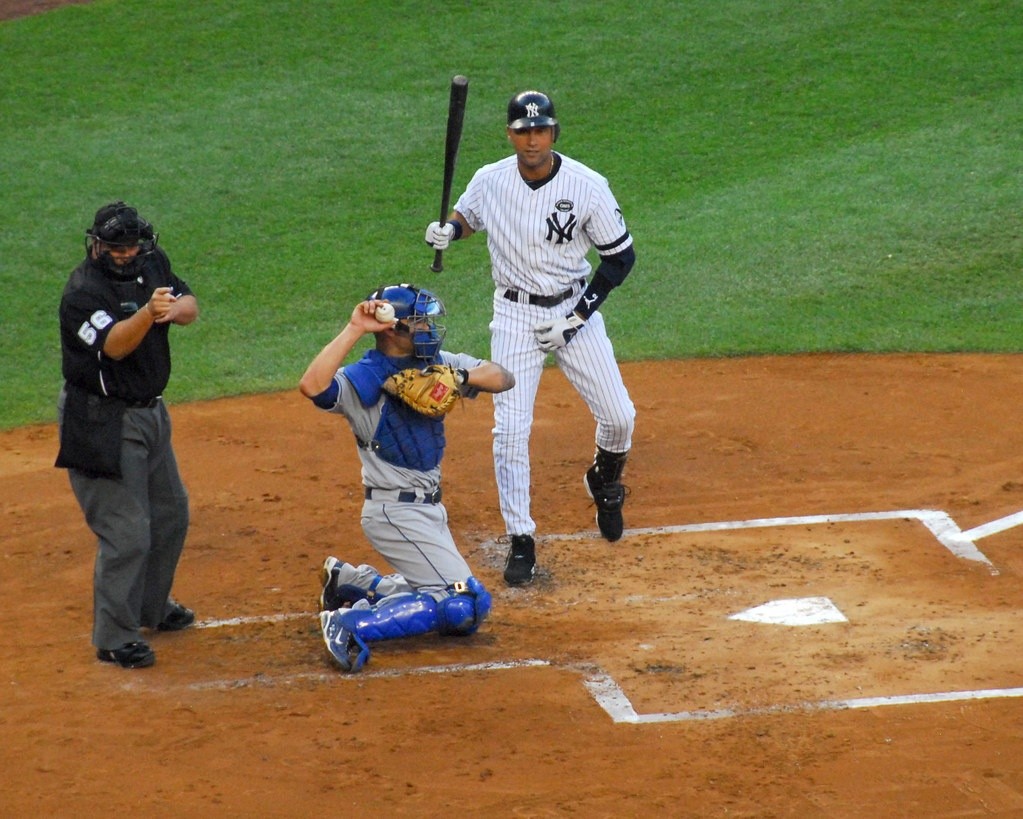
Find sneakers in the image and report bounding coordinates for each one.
[498,534,536,587]
[319,606,355,672]
[97,638,155,669]
[155,604,194,632]
[583,451,631,542]
[319,556,345,610]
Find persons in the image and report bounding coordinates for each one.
[54,201,198,668]
[426,88,637,588]
[299,282,514,672]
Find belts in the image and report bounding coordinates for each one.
[124,394,164,410]
[504,279,586,309]
[364,488,441,505]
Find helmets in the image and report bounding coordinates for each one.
[365,282,443,318]
[507,89,559,143]
[89,202,146,246]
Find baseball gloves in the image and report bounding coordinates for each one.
[380,361,465,419]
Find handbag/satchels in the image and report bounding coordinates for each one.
[52,385,124,480]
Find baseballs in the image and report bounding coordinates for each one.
[374,303,396,323]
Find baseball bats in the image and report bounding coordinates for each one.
[429,74,469,272]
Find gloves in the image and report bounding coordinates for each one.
[533,311,586,352]
[425,219,455,249]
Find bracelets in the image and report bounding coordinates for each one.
[457,367,469,385]
